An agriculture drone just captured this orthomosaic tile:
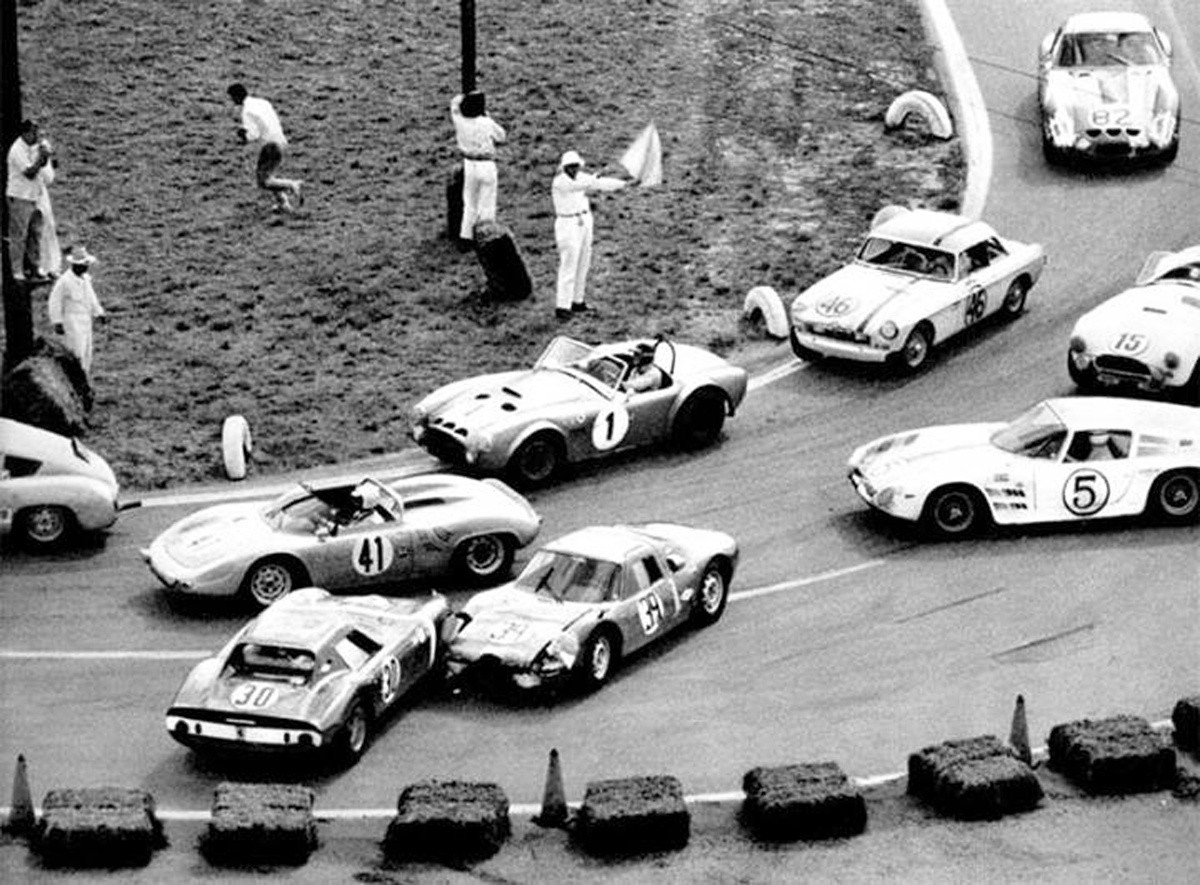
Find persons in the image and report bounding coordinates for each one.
[309,483,385,537]
[227,81,305,215]
[1047,429,1113,465]
[47,247,106,387]
[550,150,642,319]
[605,343,662,395]
[5,119,56,282]
[935,251,972,281]
[448,90,506,252]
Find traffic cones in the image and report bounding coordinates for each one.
[1008,692,1041,771]
[530,749,580,829]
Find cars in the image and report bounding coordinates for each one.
[0,417,143,550]
[1035,10,1183,173]
[790,207,1043,376]
[1067,245,1200,407]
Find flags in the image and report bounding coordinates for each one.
[620,124,663,189]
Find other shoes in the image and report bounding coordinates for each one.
[555,308,578,319]
[292,180,304,197]
[274,191,289,208]
[37,267,59,279]
[13,272,25,282]
[573,301,597,313]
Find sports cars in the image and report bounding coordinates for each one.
[165,585,453,774]
[402,334,748,492]
[141,466,543,616]
[845,394,1200,543]
[441,520,739,693]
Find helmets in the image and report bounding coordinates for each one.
[629,344,654,366]
[352,482,380,509]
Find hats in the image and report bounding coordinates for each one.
[560,150,585,170]
[66,247,97,265]
